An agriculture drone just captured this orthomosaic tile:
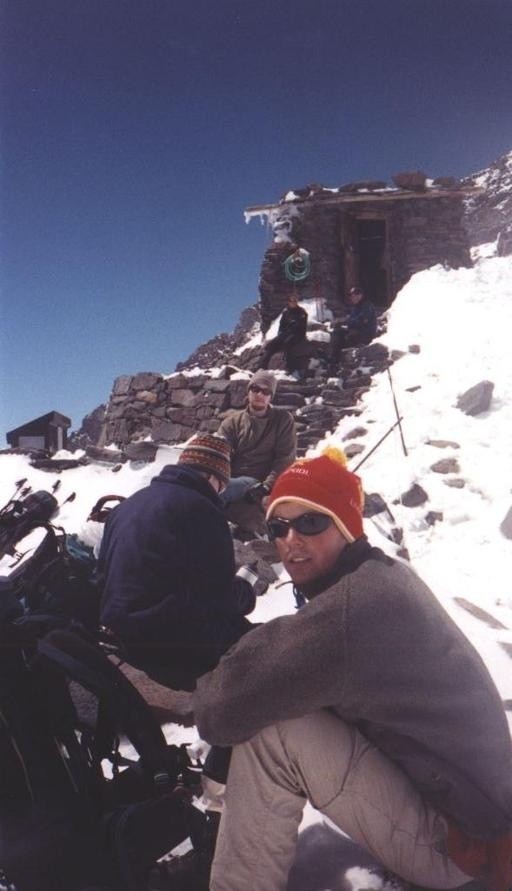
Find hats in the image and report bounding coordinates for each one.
[247,369,277,399]
[177,435,232,482]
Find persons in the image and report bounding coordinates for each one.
[245,293,309,375]
[211,366,302,596]
[185,446,511,891]
[316,284,380,378]
[88,425,271,815]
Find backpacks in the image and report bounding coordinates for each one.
[0,612,188,891]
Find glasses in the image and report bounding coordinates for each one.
[249,385,272,395]
[267,511,331,537]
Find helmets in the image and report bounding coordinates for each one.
[264,445,364,544]
